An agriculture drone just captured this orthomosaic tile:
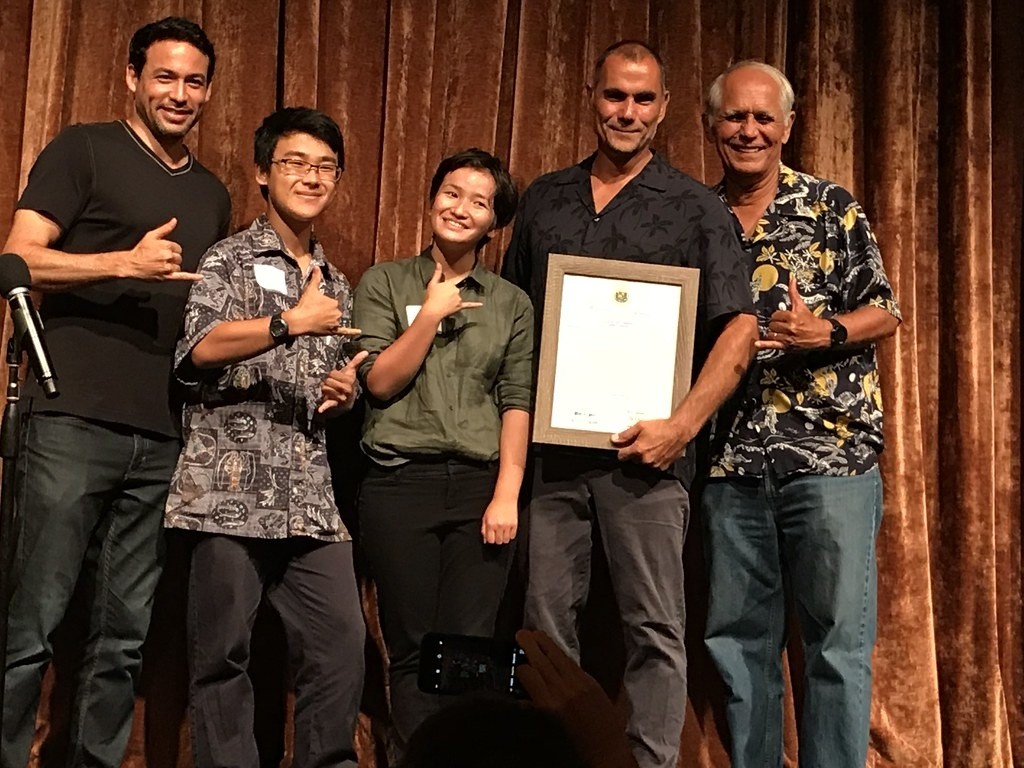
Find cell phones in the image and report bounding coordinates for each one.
[417,631,533,701]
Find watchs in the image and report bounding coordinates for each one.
[269,310,289,344]
[825,316,848,348]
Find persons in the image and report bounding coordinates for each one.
[184,106,366,768]
[346,146,535,768]
[695,61,901,765]
[501,38,758,768]
[1,11,231,768]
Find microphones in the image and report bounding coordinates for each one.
[0,252,61,399]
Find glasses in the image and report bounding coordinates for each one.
[271,159,343,182]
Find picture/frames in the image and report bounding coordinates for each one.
[529,253,701,459]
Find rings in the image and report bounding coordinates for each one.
[772,332,778,342]
